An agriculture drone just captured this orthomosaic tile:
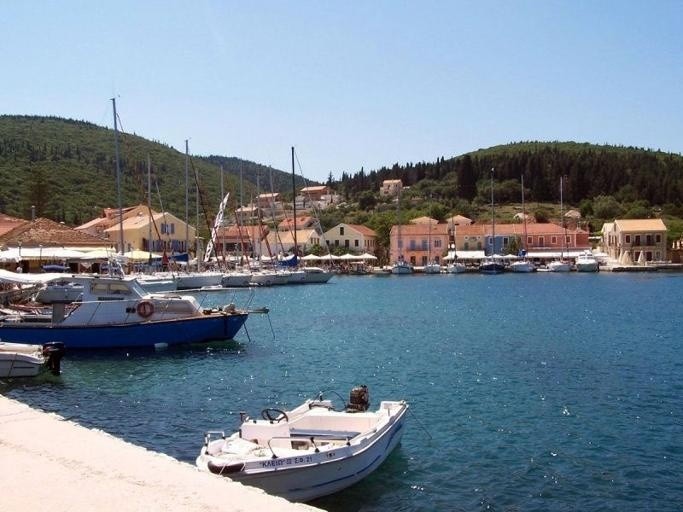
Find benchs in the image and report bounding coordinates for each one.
[288,415,372,444]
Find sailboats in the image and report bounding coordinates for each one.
[510,175,538,273]
[480,167,507,276]
[548,177,571,271]
[99,137,338,289]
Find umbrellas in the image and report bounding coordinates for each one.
[337,253,357,264]
[320,253,340,263]
[299,253,320,266]
[356,252,377,262]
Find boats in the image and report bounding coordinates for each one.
[576,250,599,271]
[0,342,63,379]
[0,273,255,357]
[425,266,436,274]
[195,385,410,502]
[391,261,416,274]
[447,263,466,274]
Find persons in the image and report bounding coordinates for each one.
[16,263,25,273]
[304,260,374,274]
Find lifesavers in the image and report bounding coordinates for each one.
[137,301,155,317]
